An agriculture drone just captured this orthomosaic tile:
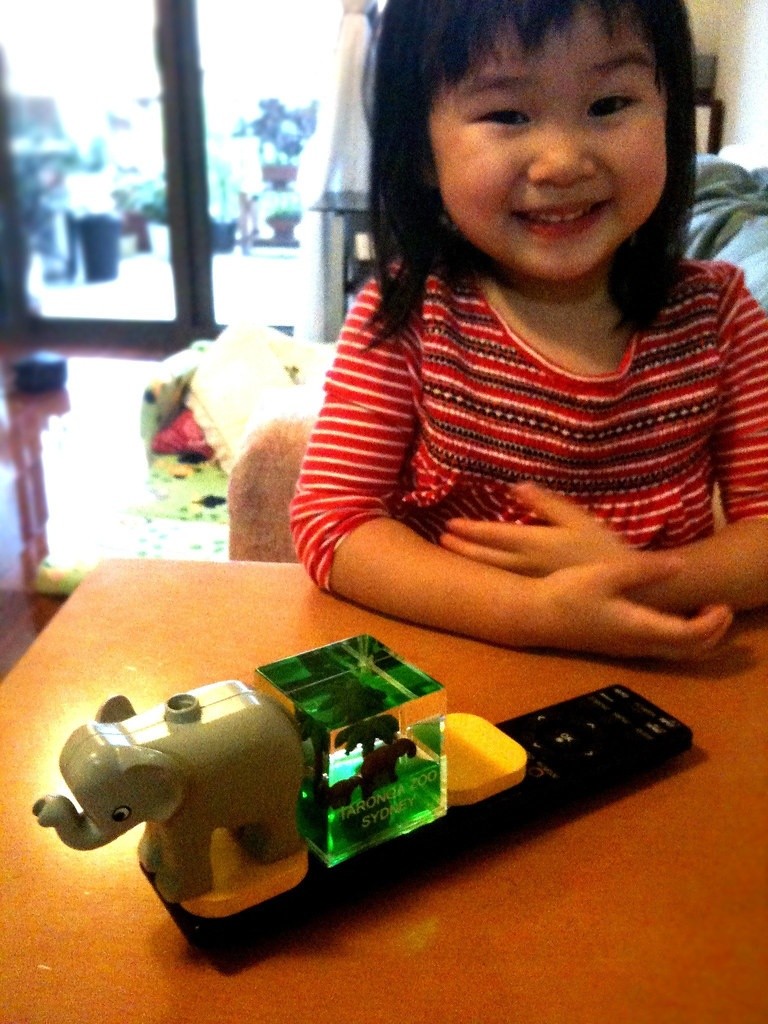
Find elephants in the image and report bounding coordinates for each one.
[31,678,306,905]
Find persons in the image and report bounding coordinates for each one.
[287,0,768,661]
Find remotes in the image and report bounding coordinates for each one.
[140,684,695,963]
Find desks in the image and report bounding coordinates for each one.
[310,191,376,344]
[0,558,768,1024]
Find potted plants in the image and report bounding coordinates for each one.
[232,98,319,249]
